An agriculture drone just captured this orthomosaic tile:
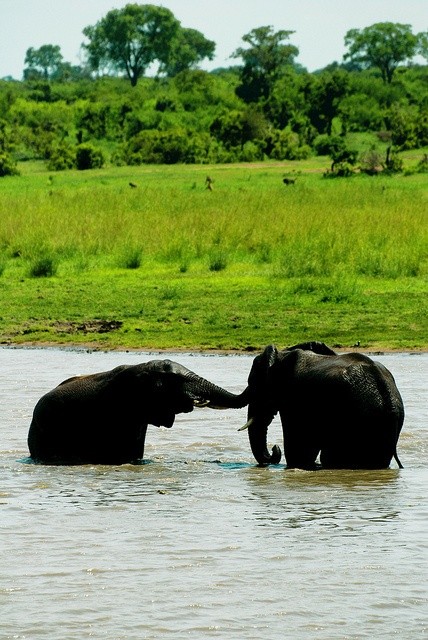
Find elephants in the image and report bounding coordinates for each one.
[26,358,265,467]
[236,340,405,471]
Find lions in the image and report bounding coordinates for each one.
[282,177,298,187]
